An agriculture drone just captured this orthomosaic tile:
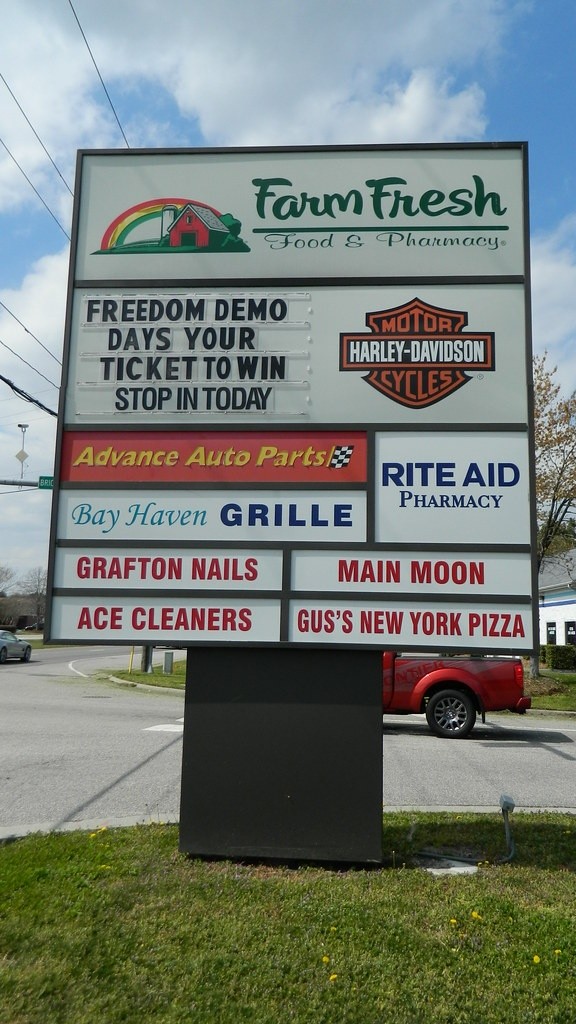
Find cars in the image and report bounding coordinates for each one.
[25,622,45,631]
[0,630,31,664]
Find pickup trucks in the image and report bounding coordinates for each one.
[381,649,531,739]
